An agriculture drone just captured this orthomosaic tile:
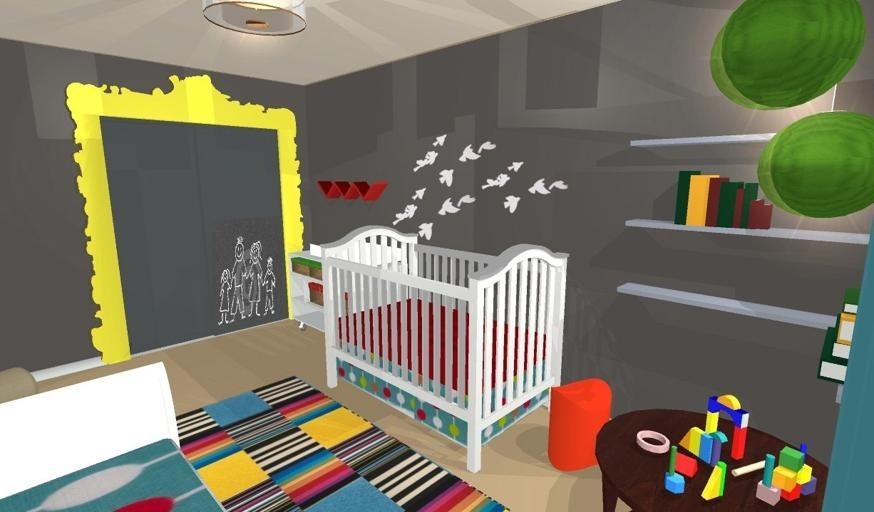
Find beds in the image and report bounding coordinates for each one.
[310,225,570,474]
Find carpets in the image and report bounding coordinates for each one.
[175,378,522,512]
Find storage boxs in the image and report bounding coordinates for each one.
[308,282,349,306]
[292,257,324,280]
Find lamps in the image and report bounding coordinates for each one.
[199,0,307,36]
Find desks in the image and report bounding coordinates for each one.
[596,408,829,512]
[0,440,230,512]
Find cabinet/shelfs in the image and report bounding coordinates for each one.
[615,131,873,332]
[289,250,407,335]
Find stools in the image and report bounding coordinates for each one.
[547,377,612,472]
